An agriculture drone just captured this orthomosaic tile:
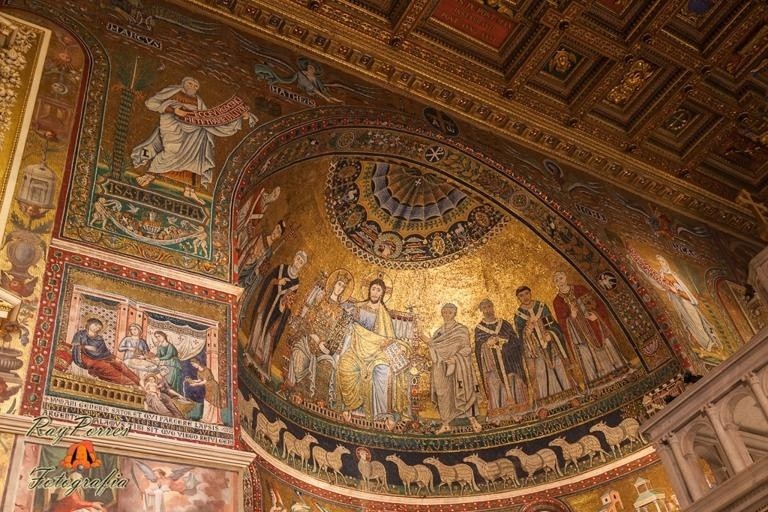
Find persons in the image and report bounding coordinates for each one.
[70,317,223,424]
[289,64,346,105]
[142,469,175,512]
[51,465,106,512]
[130,76,259,205]
[90,198,208,258]
[656,254,724,352]
